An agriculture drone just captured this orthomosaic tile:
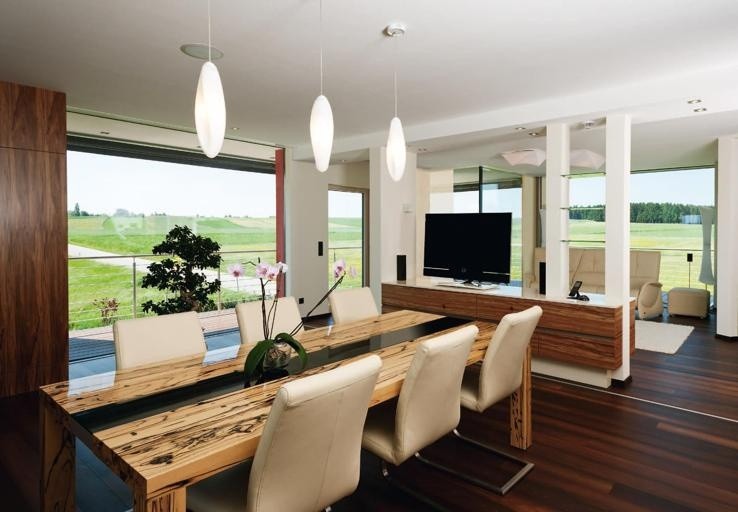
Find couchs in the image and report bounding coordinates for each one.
[525,247,665,320]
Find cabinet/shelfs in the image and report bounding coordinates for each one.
[382,283,635,370]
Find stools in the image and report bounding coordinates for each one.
[668,287,710,319]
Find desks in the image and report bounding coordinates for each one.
[37,310,532,511]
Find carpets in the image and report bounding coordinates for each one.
[634,319,694,355]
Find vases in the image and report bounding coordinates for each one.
[259,340,292,369]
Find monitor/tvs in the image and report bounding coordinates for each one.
[423,212,512,289]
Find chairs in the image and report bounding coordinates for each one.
[416,304,542,494]
[330,324,479,511]
[187,355,382,511]
[113,310,206,370]
[328,286,376,324]
[235,296,303,344]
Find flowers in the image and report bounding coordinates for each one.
[227,256,361,382]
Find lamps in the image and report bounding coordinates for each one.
[501,150,546,167]
[569,150,606,171]
[195,0,226,159]
[384,24,407,182]
[309,0,334,173]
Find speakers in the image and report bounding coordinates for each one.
[539,262,545,294]
[396,254,406,280]
